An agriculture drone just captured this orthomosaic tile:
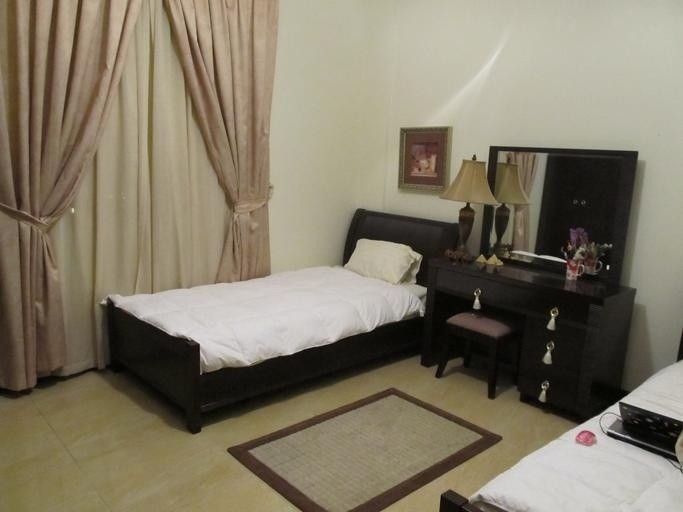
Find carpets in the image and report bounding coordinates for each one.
[227,386,502,510]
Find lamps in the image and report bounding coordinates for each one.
[493,157,532,257]
[439,152,500,261]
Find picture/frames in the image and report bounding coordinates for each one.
[396,124,455,193]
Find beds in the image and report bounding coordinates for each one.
[96,206,457,437]
[437,330,682,511]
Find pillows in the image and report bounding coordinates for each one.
[343,234,418,287]
[406,248,423,285]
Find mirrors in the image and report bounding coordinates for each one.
[478,144,641,287]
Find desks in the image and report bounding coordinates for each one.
[419,254,639,424]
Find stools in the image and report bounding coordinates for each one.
[434,307,515,401]
